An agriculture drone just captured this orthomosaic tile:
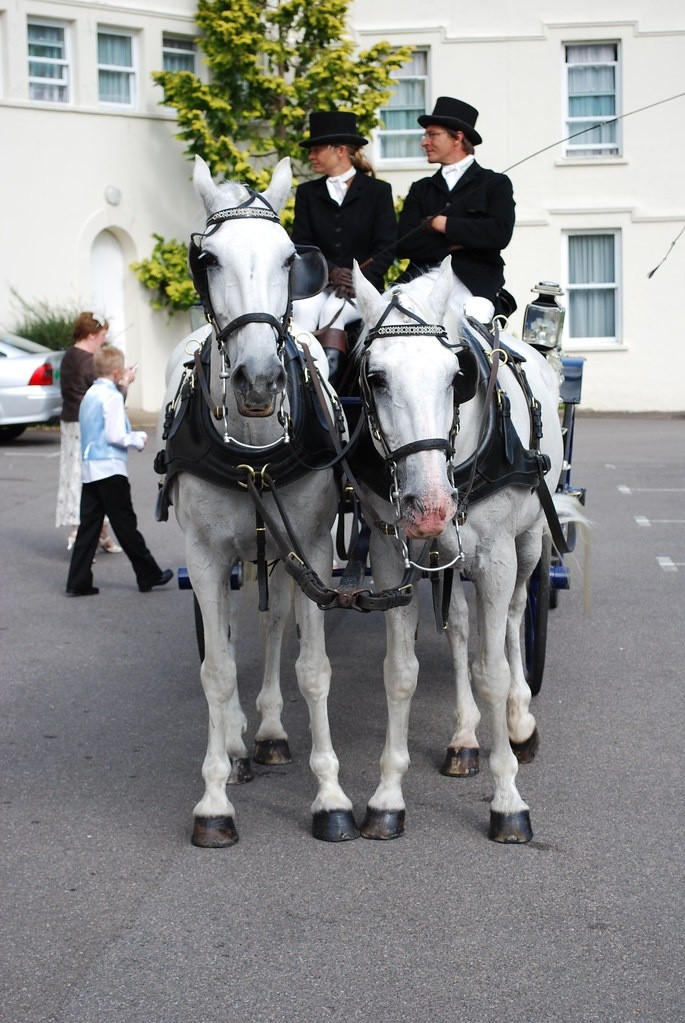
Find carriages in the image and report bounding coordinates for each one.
[145,153,588,850]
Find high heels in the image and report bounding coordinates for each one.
[95,535,123,554]
[67,531,96,562]
[66,584,100,596]
[139,569,174,593]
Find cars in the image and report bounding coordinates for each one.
[0,330,66,442]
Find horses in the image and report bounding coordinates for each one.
[159,151,593,852]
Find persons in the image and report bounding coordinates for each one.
[394,96,517,332]
[289,112,399,382]
[57,311,175,597]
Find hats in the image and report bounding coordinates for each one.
[298,112,368,148]
[418,96,482,146]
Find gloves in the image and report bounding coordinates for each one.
[419,215,435,231]
[334,285,356,299]
[328,268,354,288]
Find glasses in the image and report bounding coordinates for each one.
[305,147,322,155]
[420,131,450,140]
